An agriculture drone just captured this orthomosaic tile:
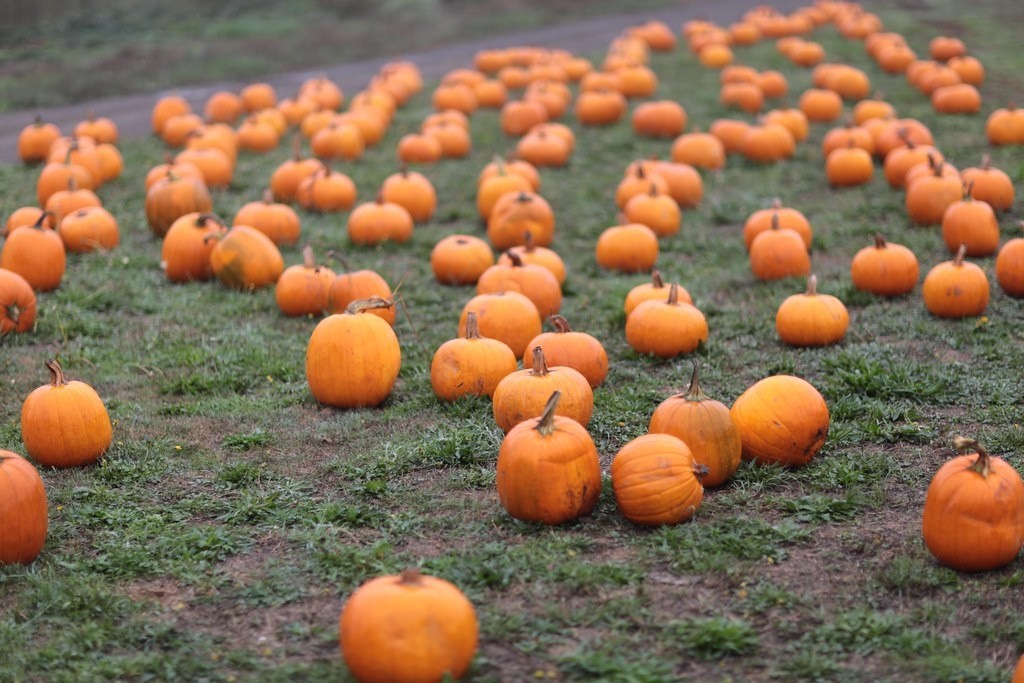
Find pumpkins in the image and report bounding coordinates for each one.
[0,1,1023,683]
